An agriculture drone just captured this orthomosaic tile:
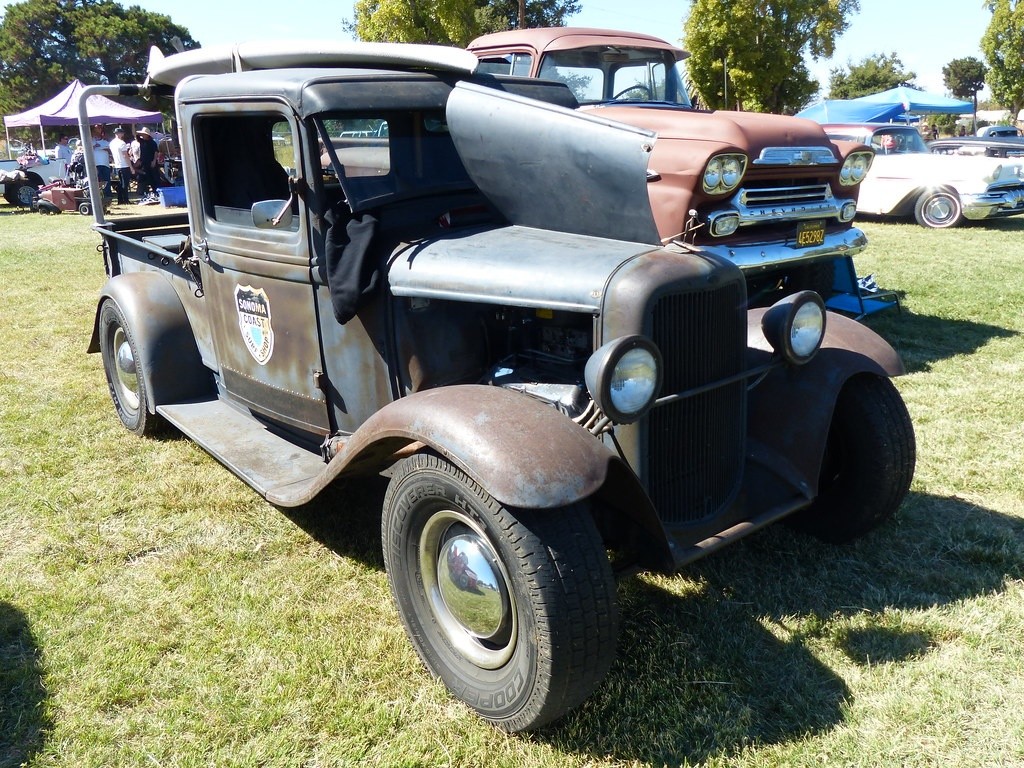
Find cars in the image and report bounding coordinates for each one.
[272,130,285,146]
[0,138,27,155]
[78,39,917,736]
[68,138,78,148]
[819,123,1024,229]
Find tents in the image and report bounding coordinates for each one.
[3,78,172,162]
[793,86,977,146]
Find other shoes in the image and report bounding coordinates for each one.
[117,202,123,204]
[124,201,135,205]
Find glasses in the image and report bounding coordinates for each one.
[62,137,68,140]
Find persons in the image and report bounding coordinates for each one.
[959,125,965,136]
[881,136,897,150]
[108,126,135,205]
[54,133,73,165]
[75,140,84,153]
[924,125,928,131]
[128,127,171,198]
[90,133,111,214]
[932,125,937,140]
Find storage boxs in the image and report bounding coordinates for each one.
[156,186,186,207]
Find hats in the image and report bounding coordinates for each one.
[136,127,151,136]
[113,128,127,134]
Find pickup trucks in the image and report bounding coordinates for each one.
[317,26,874,308]
[0,158,67,208]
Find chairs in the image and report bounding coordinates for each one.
[218,136,299,215]
